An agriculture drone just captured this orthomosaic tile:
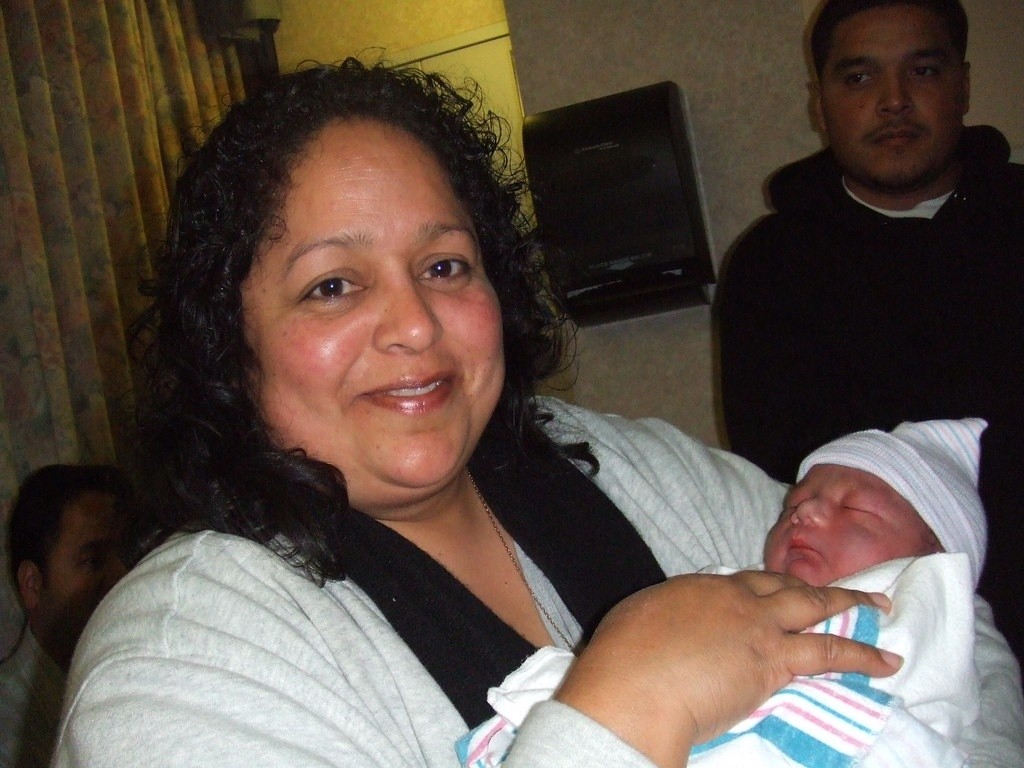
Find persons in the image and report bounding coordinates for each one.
[462,418,988,768]
[53,61,1024,768]
[0,465,134,768]
[713,1,1024,680]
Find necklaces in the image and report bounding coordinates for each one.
[463,464,580,660]
